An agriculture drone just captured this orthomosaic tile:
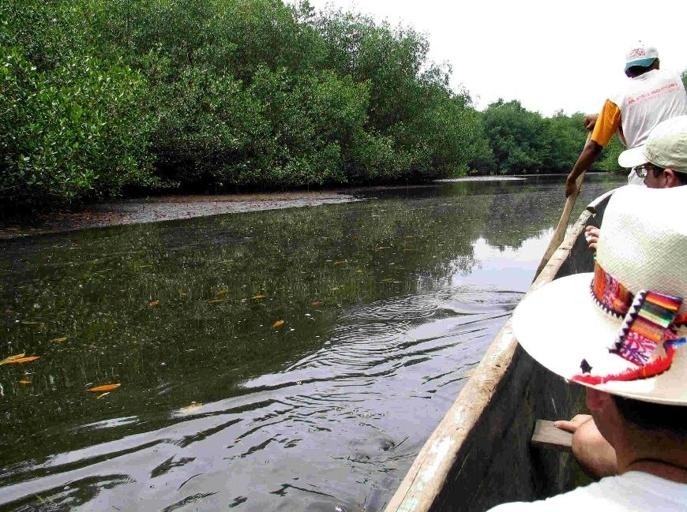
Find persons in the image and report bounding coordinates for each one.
[583,116,687,250]
[484,184,687,512]
[565,38,687,199]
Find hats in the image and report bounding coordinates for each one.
[618,113,687,174]
[625,42,658,72]
[512,184,687,407]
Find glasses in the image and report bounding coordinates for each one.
[634,164,658,178]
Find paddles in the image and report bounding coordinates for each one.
[534,170,585,281]
